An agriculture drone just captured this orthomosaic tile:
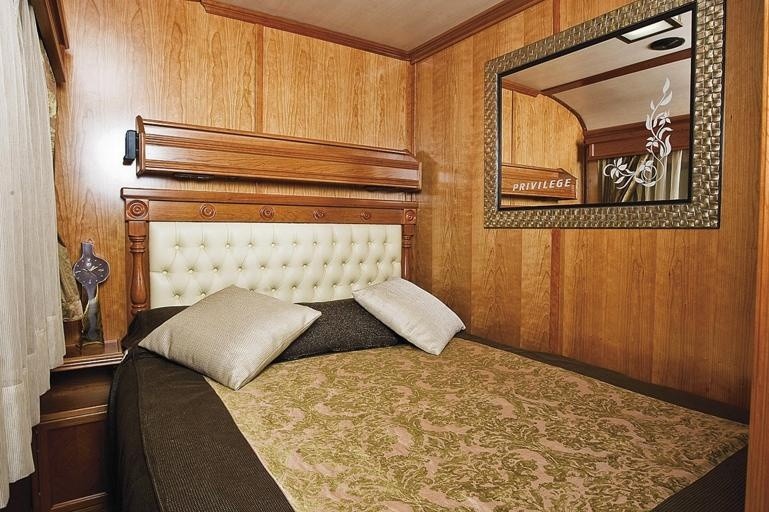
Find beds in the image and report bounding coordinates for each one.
[103,187,748,512]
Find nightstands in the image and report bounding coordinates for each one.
[25,337,126,511]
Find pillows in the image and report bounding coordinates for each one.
[138,283,322,391]
[121,298,405,361]
[352,275,467,357]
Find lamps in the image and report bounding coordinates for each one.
[615,15,685,45]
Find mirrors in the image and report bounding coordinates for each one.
[483,1,725,229]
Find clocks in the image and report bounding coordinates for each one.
[73,240,111,349]
